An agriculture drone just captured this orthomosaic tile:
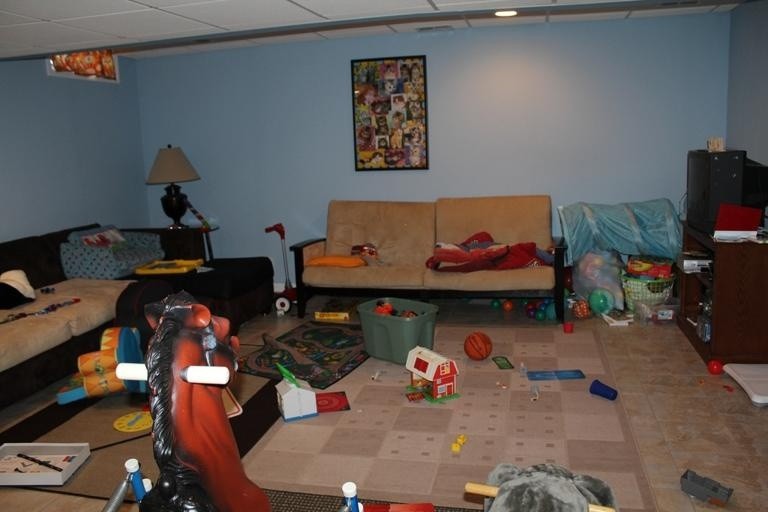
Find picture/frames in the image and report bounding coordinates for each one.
[351,55,429,171]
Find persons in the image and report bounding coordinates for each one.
[355,60,426,170]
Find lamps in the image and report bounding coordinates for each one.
[145,144,202,229]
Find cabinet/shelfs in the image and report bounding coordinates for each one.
[676,219,768,368]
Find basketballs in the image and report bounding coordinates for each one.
[464,332,492,360]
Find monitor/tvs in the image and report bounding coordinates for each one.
[686,148,768,231]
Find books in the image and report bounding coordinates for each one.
[600,308,635,328]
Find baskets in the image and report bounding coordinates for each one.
[620,274,676,313]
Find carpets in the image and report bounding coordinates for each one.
[236,321,369,391]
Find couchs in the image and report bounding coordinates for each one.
[289,195,568,323]
[0,224,274,412]
